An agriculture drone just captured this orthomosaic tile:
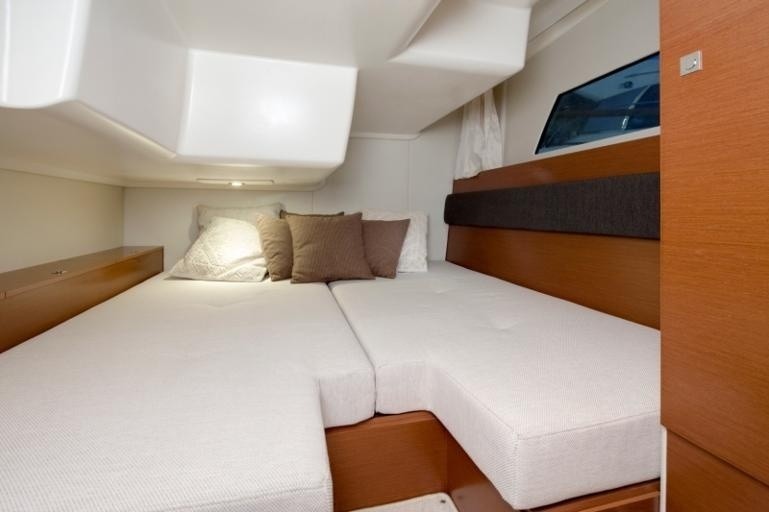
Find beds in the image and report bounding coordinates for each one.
[0,260,667,512]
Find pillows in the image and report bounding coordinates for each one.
[285,211,375,283]
[196,202,283,236]
[360,218,411,279]
[255,213,293,281]
[361,209,429,273]
[279,209,344,219]
[167,216,269,282]
